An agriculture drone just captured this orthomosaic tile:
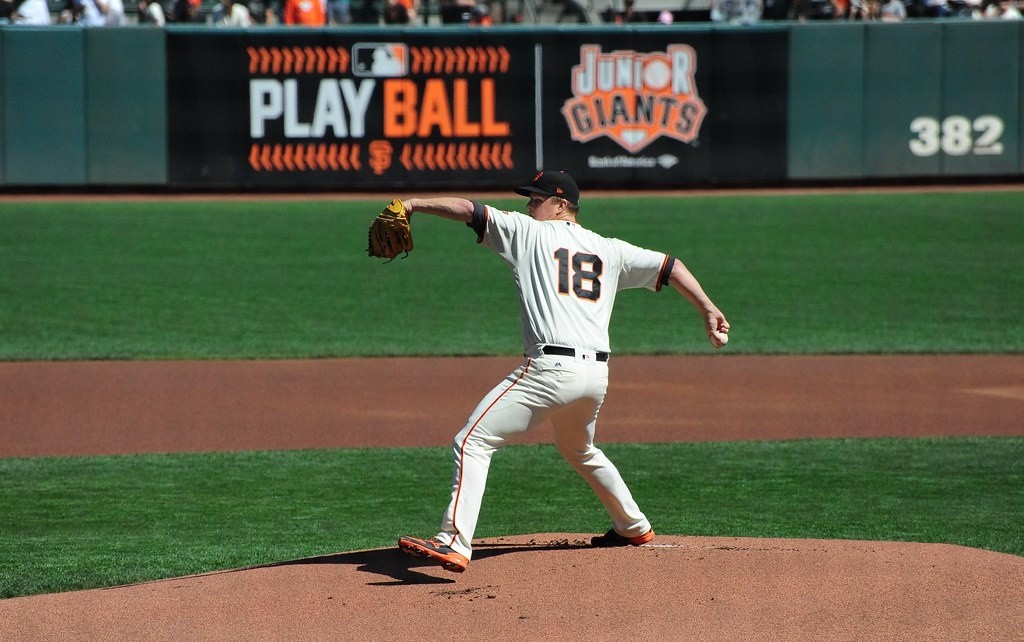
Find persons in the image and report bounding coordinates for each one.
[368,168,731,573]
[0,0,1024,29]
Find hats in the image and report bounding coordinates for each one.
[514,170,580,205]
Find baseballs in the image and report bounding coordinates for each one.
[710,330,729,348]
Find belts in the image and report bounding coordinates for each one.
[542,345,609,363]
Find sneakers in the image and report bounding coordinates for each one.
[590,528,655,547]
[398,535,469,573]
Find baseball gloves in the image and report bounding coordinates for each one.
[367,198,414,259]
